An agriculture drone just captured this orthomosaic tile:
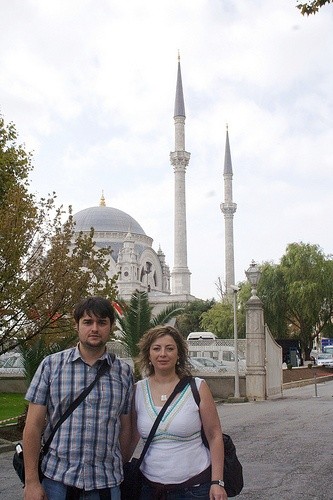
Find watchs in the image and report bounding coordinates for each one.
[211,479,224,488]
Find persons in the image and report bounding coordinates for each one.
[129,324,228,500]
[21,297,134,500]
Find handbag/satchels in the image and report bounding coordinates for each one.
[11,443,44,487]
[211,432,246,498]
[116,459,141,499]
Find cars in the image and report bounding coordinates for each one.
[315,346,333,368]
[187,357,233,375]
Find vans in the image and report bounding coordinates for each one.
[187,332,217,341]
[188,345,247,374]
